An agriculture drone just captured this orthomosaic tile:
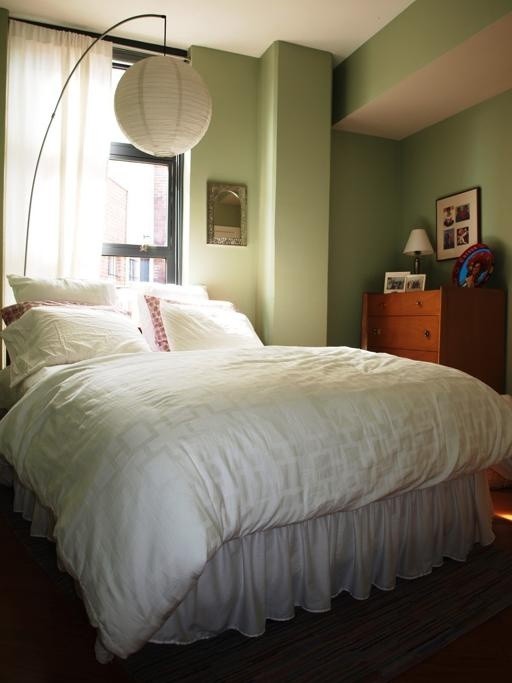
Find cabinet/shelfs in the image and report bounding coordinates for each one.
[360,288,506,394]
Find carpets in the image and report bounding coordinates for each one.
[120,518,512,683]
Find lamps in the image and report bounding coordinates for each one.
[403,226,432,273]
[24,14,214,270]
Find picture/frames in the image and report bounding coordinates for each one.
[383,270,426,293]
[436,186,481,261]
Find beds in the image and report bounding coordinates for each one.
[1,282,512,662]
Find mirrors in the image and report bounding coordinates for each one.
[209,182,248,246]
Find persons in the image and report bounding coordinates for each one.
[466,261,481,284]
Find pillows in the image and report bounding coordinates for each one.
[1,273,262,378]
